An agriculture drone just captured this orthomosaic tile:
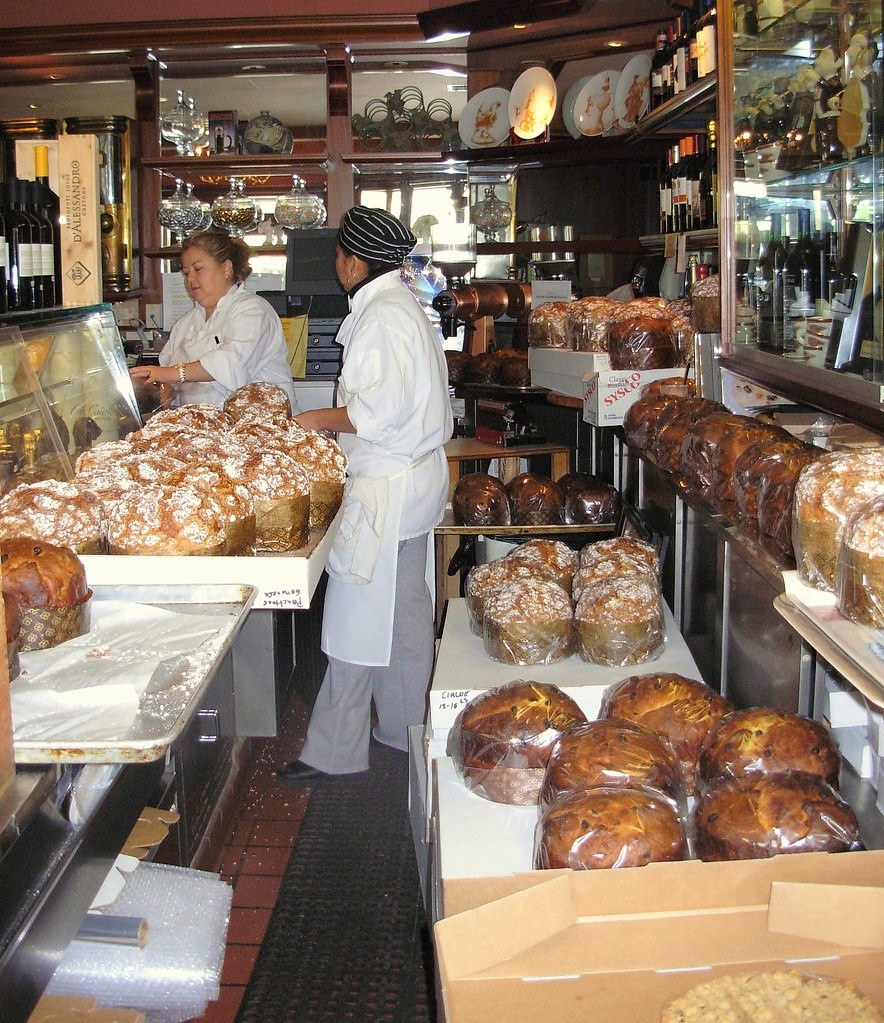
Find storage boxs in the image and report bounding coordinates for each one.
[75,484,352,611]
[408,568,884,1022]
[519,348,694,428]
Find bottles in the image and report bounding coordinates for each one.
[649,0,717,113]
[244,111,287,154]
[660,121,718,231]
[470,184,511,242]
[685,206,838,359]
[157,176,326,246]
[0,146,61,311]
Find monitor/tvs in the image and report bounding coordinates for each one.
[286,230,349,296]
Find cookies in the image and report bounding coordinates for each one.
[659,969,884,1023]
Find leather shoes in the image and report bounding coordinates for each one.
[278,762,327,780]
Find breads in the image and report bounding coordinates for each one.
[447,671,860,871]
[0,381,348,683]
[442,348,531,387]
[624,375,884,632]
[452,473,620,525]
[527,274,721,371]
[465,538,664,666]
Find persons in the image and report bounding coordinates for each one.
[280,207,453,780]
[128,232,302,417]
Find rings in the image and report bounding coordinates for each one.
[147,370,151,376]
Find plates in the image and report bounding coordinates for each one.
[458,55,653,149]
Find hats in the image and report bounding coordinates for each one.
[340,206,417,267]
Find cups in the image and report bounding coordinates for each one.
[659,385,688,397]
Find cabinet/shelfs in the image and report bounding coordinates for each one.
[137,70,716,256]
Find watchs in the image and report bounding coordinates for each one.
[177,362,186,382]
